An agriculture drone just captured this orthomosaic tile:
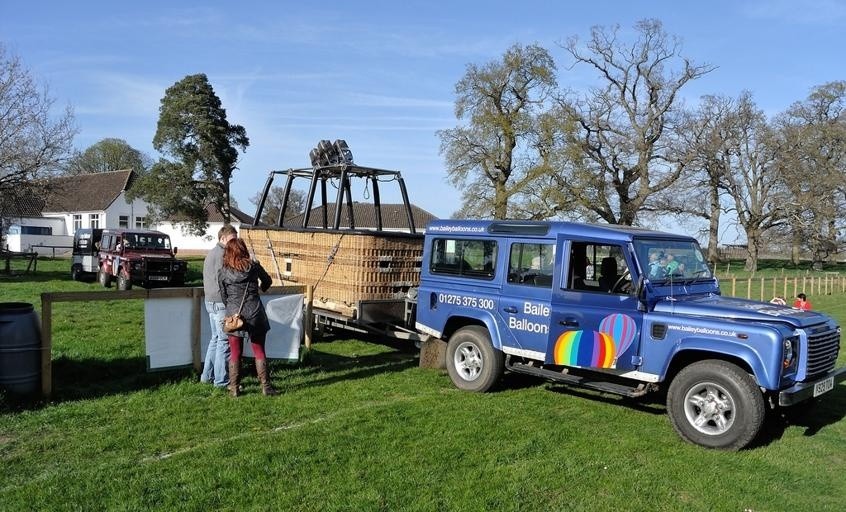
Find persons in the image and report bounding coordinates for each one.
[217,238,285,397]
[794,294,810,311]
[769,296,787,308]
[201,225,243,391]
[648,249,685,279]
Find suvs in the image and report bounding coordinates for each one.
[95,230,190,292]
[414,217,838,452]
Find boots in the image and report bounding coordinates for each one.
[228,362,241,397]
[255,360,280,395]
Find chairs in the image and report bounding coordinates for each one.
[598,257,624,292]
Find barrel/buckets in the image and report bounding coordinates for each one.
[0,303,43,394]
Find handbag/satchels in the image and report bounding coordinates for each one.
[223,314,245,332]
